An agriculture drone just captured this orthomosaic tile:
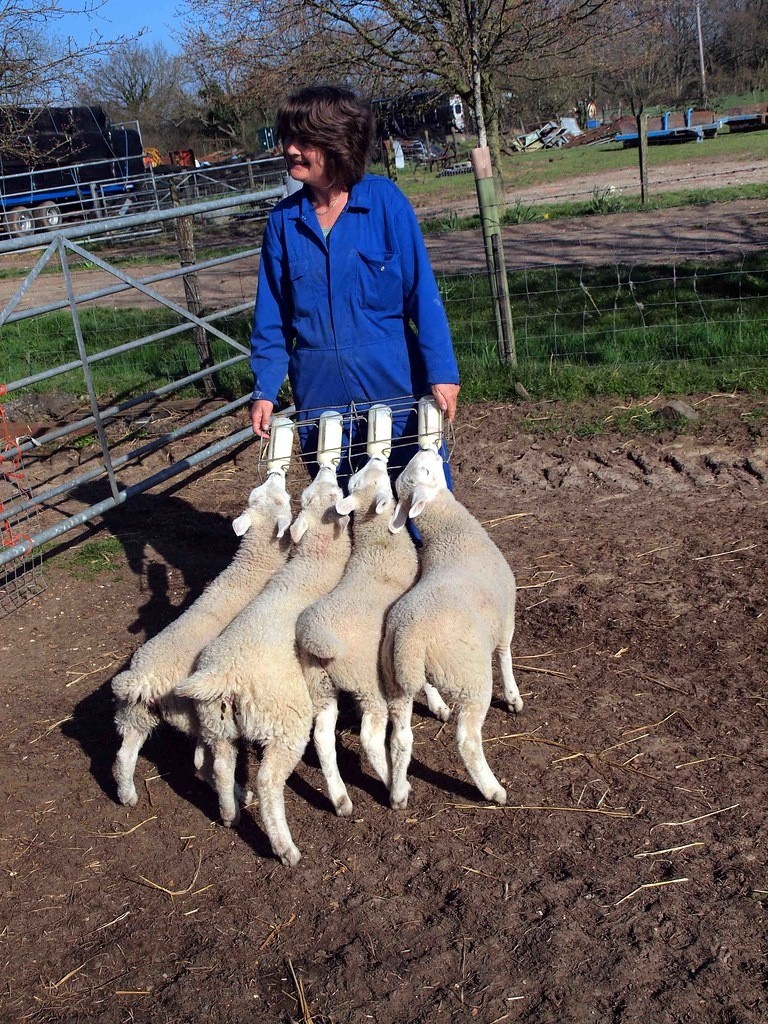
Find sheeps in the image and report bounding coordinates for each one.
[295,456,422,817]
[176,464,353,867]
[377,447,526,811]
[108,463,291,827]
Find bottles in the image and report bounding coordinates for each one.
[367,403,393,463]
[267,418,295,476]
[317,411,343,471]
[418,395,442,452]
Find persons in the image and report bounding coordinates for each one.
[247,87,460,548]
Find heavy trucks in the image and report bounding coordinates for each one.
[0,106,146,240]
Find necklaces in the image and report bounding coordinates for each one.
[316,189,342,215]
